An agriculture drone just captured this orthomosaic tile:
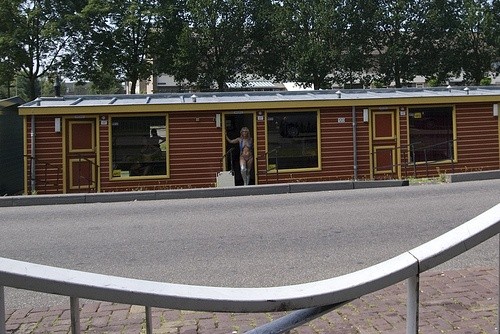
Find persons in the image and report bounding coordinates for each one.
[225,126,254,185]
[148,128,166,173]
[129,134,160,175]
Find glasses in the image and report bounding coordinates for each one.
[241,130,248,133]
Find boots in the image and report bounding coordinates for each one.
[246,170,252,187]
[241,165,247,187]
